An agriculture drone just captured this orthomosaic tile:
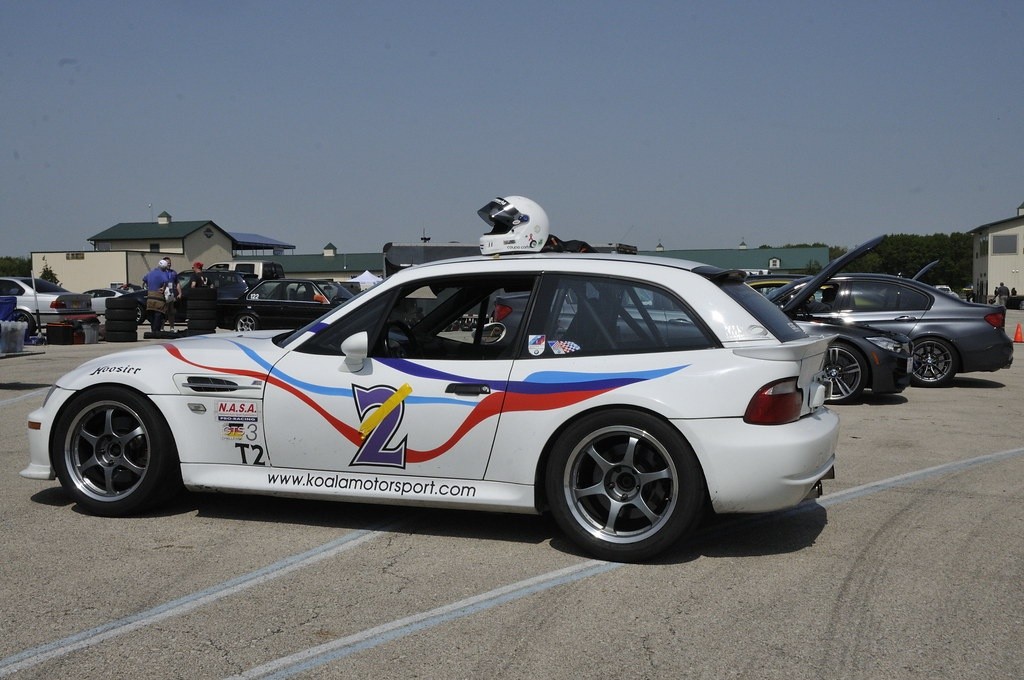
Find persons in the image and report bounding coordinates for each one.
[968,290,975,302]
[1011,288,1017,297]
[191,263,214,288]
[143,256,182,332]
[994,282,1010,309]
[805,288,836,312]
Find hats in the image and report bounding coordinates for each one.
[158,260,168,267]
[194,261,203,269]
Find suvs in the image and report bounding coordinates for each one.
[121,268,249,326]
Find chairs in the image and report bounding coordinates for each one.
[290,288,311,301]
[556,297,601,351]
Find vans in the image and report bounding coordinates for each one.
[206,260,285,281]
[934,284,951,293]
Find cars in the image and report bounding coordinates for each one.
[218,279,357,334]
[83,289,133,315]
[0,277,97,335]
[19,253,841,565]
[760,273,1015,389]
[495,233,915,402]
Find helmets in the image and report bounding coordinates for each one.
[477,194,549,255]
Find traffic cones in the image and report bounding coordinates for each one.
[1011,322,1024,343]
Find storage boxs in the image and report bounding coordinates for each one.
[45,322,99,344]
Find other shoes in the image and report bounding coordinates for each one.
[170,328,179,332]
